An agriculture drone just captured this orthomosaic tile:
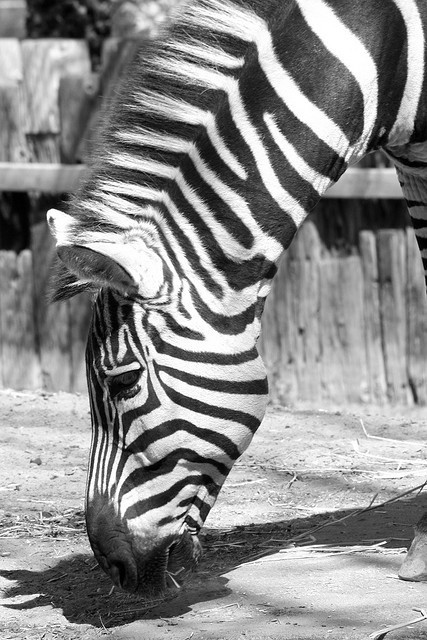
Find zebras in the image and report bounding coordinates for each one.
[40,0,427,600]
